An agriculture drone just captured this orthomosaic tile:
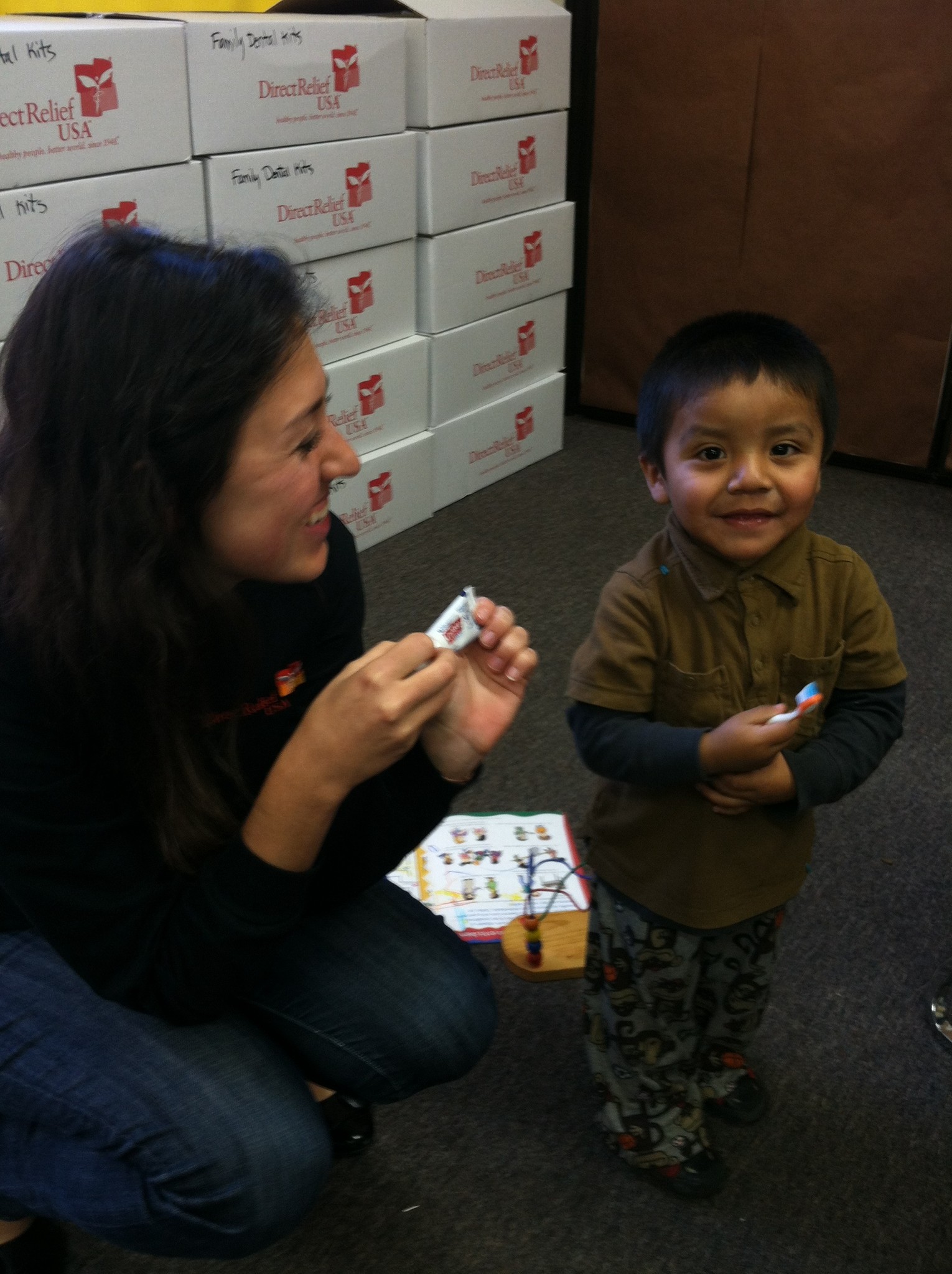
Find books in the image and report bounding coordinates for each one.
[385,809,591,944]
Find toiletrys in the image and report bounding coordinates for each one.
[414,586,480,672]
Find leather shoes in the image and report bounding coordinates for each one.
[313,1090,376,1159]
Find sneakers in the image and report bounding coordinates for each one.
[703,1065,770,1123]
[636,1146,732,1200]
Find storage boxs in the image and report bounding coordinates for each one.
[0,0,577,556]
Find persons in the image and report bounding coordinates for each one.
[0,210,540,1273]
[566,307,907,1206]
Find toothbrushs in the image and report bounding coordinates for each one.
[767,680,822,725]
